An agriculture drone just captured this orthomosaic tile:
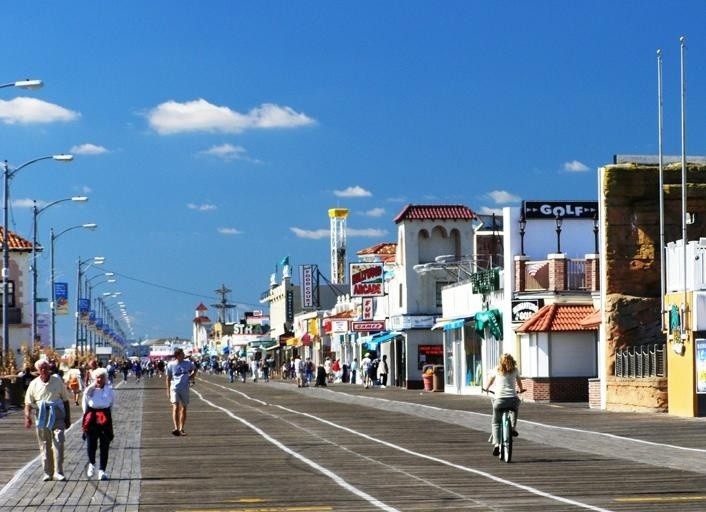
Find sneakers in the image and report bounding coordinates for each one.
[172,428,181,437]
[97,469,110,482]
[53,472,67,482]
[180,428,188,436]
[75,400,79,406]
[86,462,97,478]
[43,473,52,482]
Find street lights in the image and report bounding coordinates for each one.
[2,149,76,374]
[28,194,90,354]
[47,223,99,353]
[0,78,44,96]
[74,253,136,371]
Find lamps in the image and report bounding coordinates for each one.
[412,252,504,282]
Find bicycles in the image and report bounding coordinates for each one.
[481,387,530,463]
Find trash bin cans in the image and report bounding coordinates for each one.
[432,365,444,392]
[421,374,433,391]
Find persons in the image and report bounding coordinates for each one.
[106,360,165,384]
[48,361,108,407]
[22,367,35,392]
[486,352,522,455]
[187,351,277,384]
[81,368,115,479]
[282,352,389,388]
[165,349,198,437]
[24,359,71,480]
